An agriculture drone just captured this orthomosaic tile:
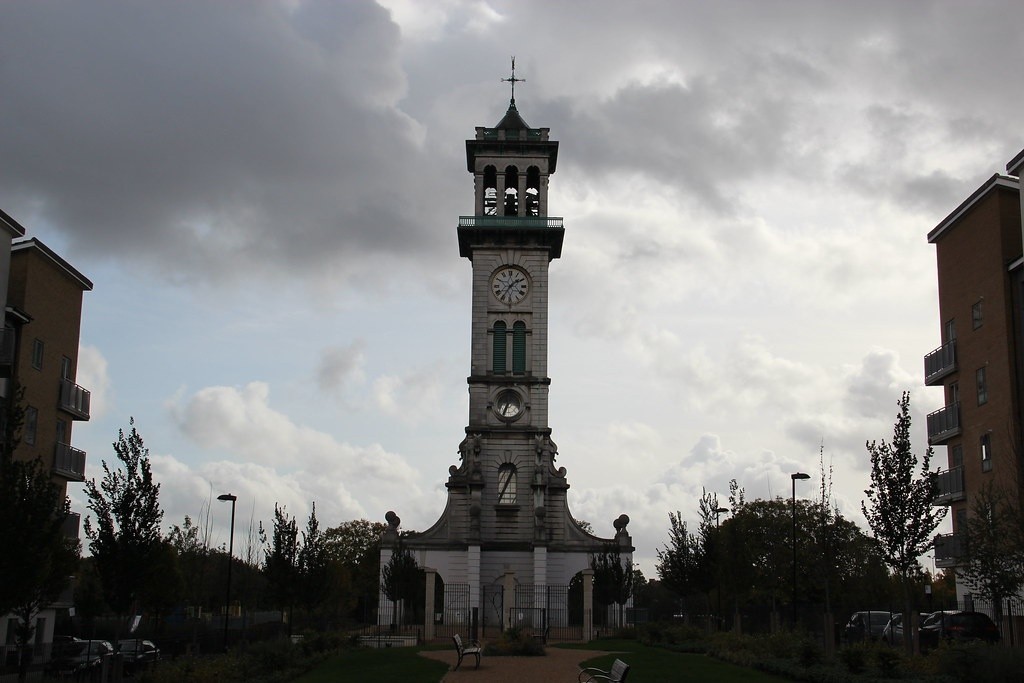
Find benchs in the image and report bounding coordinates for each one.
[451,633,482,672]
[577,658,630,683]
[531,625,551,646]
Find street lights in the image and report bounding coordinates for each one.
[791,471,811,629]
[216,493,236,652]
[714,508,729,633]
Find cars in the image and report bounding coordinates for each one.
[57,641,117,675]
[882,612,930,648]
[846,611,893,640]
[59,636,83,643]
[918,611,1000,653]
[103,639,160,667]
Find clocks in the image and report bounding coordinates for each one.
[491,268,529,305]
